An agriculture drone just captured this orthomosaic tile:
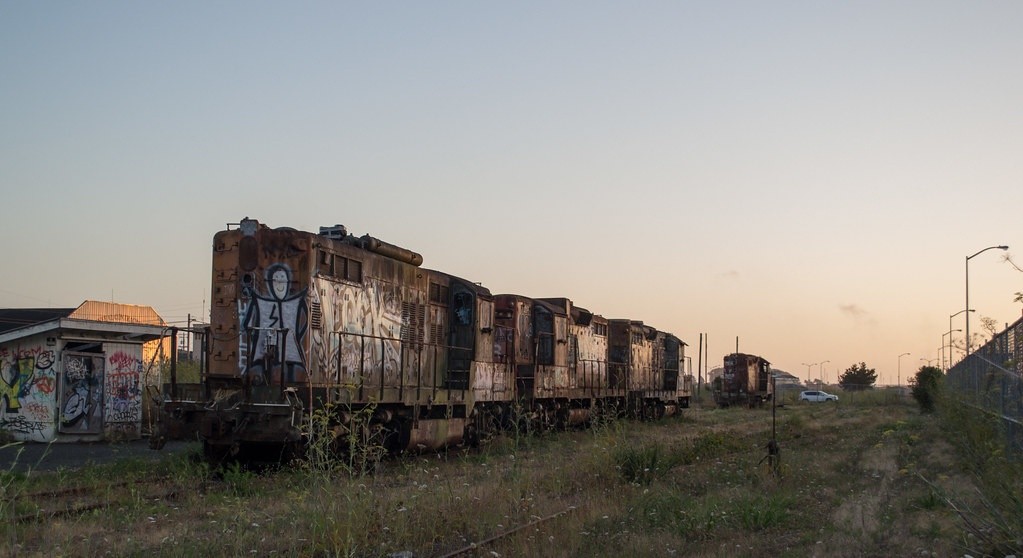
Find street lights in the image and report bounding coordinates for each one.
[801,360,830,387]
[920,244,1009,387]
[897,350,911,389]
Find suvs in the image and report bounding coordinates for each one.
[798,390,838,403]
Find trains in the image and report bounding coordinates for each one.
[141,214,694,481]
[713,353,773,410]
